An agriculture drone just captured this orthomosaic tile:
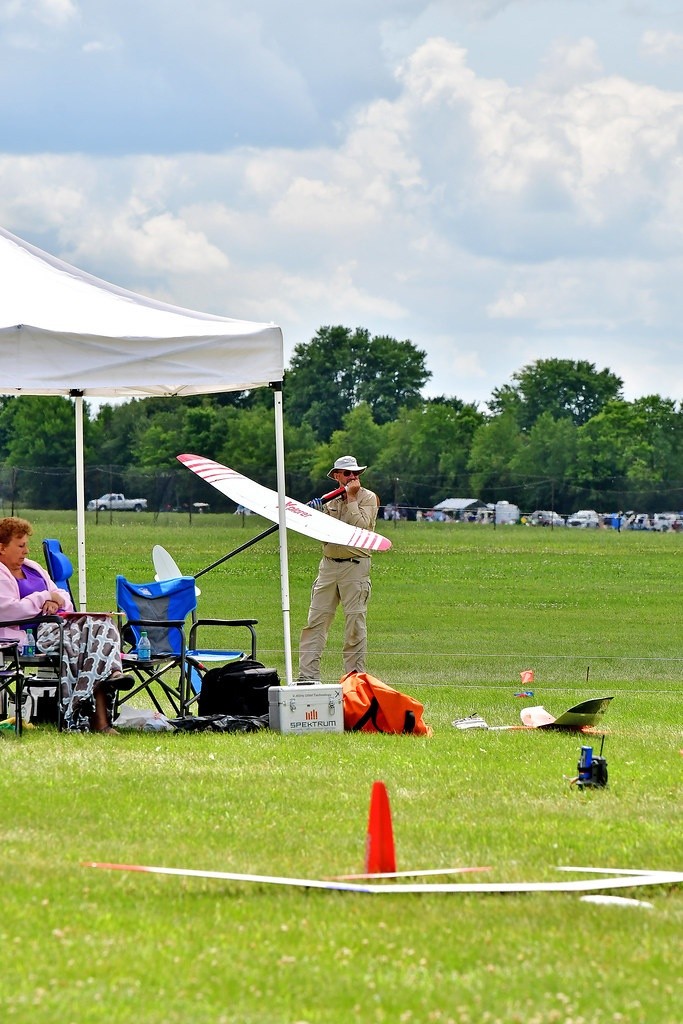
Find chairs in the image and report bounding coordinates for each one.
[113,575,257,729]
[42,538,76,611]
[0,611,111,738]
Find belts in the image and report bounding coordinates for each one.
[331,557,360,564]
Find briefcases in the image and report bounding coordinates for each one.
[197,659,281,716]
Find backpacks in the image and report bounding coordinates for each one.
[339,668,434,738]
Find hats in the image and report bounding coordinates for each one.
[326,455,367,481]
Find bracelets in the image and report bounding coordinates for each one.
[43,600,59,608]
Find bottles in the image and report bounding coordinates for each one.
[21,629,35,659]
[138,631,151,661]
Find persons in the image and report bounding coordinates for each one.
[385,504,400,520]
[299,456,380,681]
[0,517,135,734]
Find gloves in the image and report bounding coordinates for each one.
[306,498,323,512]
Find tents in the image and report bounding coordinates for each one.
[0,226,293,686]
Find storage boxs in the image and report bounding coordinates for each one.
[268,680,345,733]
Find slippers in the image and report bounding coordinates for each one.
[93,725,120,736]
[98,669,136,693]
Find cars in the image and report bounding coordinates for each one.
[383,504,408,520]
[526,511,565,527]
[604,511,683,531]
[566,510,599,528]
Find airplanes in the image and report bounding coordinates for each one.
[152,453,393,597]
[451,696,615,733]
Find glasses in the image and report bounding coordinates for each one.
[335,470,360,476]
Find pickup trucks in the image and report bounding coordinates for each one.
[87,494,148,512]
